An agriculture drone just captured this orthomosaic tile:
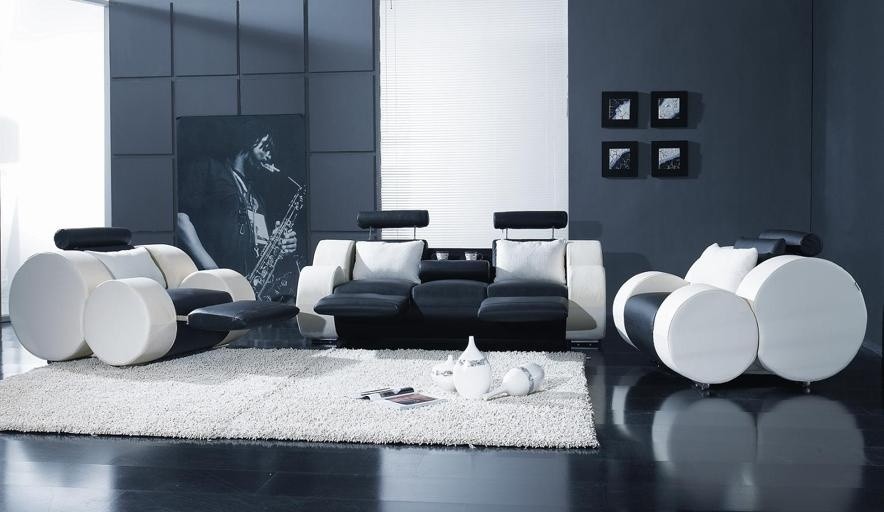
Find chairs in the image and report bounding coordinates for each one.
[612,229,868,393]
[8,226,299,367]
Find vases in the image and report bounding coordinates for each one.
[455,336,493,397]
[483,363,545,401]
[430,353,456,393]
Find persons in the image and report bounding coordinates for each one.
[175,119,298,297]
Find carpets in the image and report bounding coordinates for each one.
[0,347,602,452]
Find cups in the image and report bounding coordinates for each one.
[465,251,478,261]
[436,250,448,262]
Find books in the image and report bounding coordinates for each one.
[345,385,440,409]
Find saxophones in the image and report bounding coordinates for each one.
[246,160,307,303]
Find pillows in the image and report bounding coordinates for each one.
[685,244,757,292]
[352,241,424,283]
[493,239,567,283]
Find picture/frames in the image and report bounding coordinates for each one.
[602,90,639,129]
[649,89,689,129]
[650,140,687,179]
[602,140,639,180]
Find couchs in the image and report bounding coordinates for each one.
[295,209,606,351]
[610,368,866,509]
